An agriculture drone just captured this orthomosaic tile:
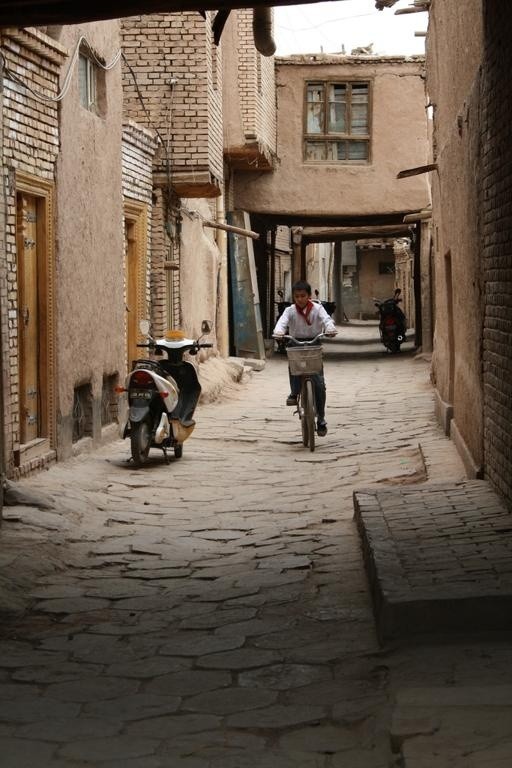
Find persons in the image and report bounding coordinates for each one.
[273,280,337,437]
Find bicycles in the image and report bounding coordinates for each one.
[272,332,335,451]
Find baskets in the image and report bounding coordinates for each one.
[286,345,323,376]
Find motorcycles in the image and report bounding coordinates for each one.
[115,319,214,465]
[277,289,295,354]
[372,288,407,353]
[315,289,336,316]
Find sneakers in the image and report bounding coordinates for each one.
[317,420,327,436]
[286,394,298,405]
